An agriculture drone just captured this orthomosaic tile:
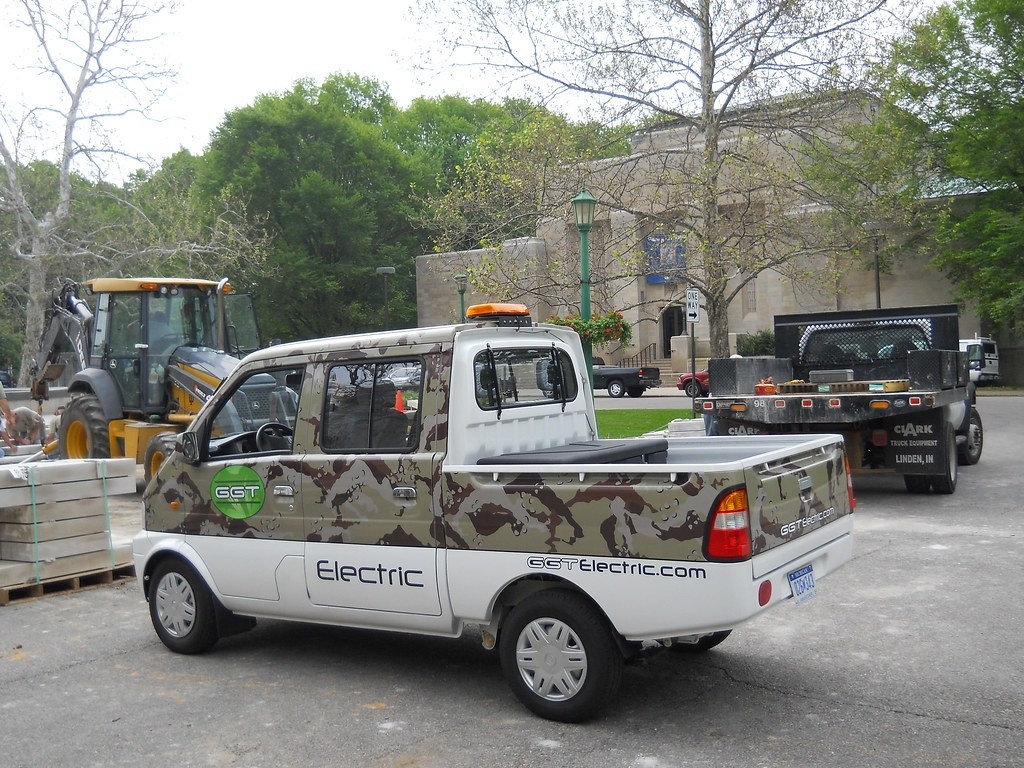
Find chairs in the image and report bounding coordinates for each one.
[815,344,845,360]
[337,379,409,448]
[890,341,918,357]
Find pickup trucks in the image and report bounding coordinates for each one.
[536,352,662,398]
[131,302,859,723]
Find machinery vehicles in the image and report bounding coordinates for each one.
[27,276,299,487]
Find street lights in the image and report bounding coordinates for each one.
[571,180,598,407]
[454,272,467,324]
[376,267,395,331]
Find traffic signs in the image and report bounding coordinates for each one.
[686,288,700,323]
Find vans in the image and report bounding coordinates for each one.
[876,332,1000,382]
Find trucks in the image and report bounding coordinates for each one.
[693,304,986,496]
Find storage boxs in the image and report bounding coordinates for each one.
[808,369,853,383]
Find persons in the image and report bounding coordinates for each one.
[0,381,66,459]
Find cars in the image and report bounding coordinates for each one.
[0,370,18,388]
[835,343,874,363]
[382,367,423,389]
[676,354,742,398]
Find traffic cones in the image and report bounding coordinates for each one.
[395,390,407,412]
